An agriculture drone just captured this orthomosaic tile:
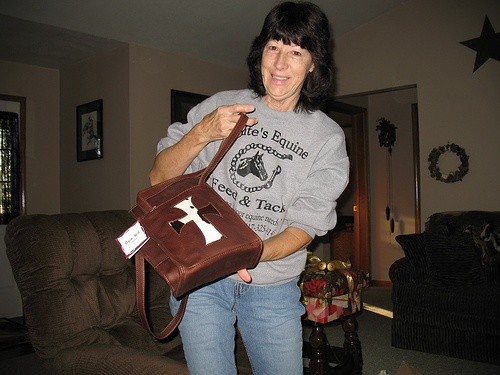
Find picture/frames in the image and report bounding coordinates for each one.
[76,98,104,162]
[171,89,210,125]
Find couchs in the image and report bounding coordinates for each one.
[389,211,500,366]
[5,210,254,375]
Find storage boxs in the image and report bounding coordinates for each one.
[302,251,362,324]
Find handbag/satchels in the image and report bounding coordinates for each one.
[127,113,264,339]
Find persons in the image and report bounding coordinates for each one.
[149,2,349,375]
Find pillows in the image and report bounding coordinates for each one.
[395,229,487,289]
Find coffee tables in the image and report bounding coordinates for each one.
[300,312,364,375]
